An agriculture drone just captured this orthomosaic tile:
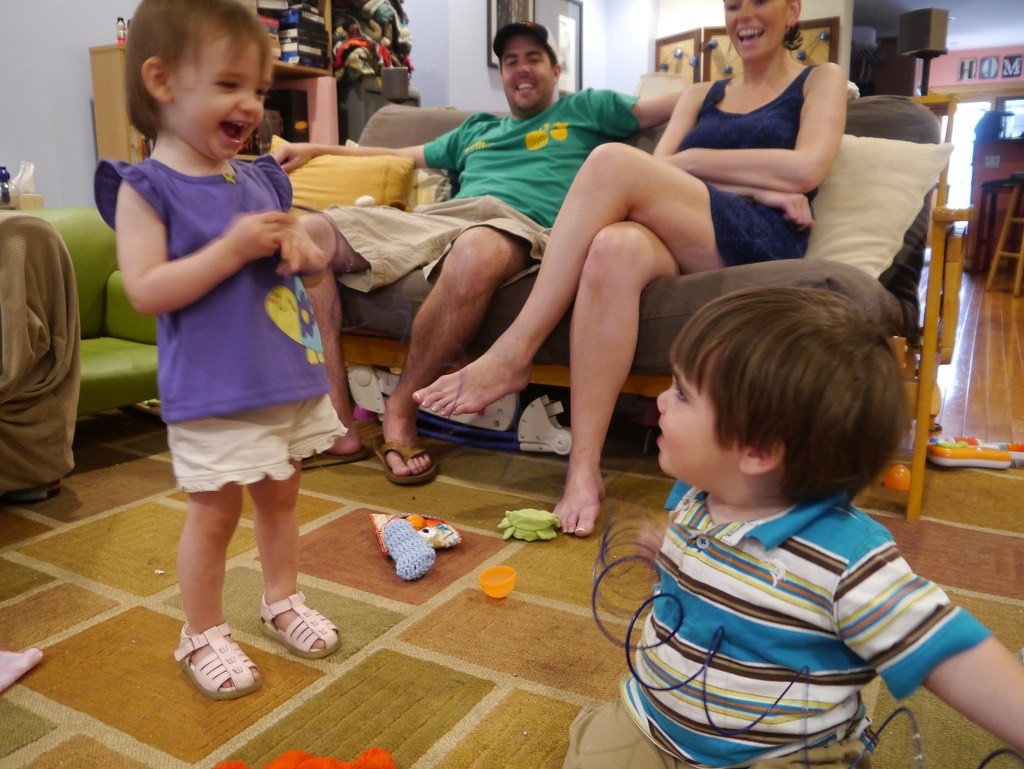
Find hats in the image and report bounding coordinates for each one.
[493,20,562,73]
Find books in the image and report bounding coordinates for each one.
[132,109,284,169]
[256,0,330,69]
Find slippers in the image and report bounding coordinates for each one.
[301,444,369,472]
[372,432,438,487]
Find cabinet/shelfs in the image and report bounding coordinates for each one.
[87,0,334,171]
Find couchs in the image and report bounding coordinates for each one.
[0,91,978,520]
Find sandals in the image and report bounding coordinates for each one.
[260,592,340,660]
[172,622,263,700]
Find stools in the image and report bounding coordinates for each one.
[972,171,1024,298]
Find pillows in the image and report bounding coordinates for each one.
[344,139,451,214]
[268,134,414,216]
[802,135,956,280]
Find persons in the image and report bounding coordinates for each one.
[93,0,343,702]
[561,285,1024,769]
[276,21,682,486]
[410,0,847,537]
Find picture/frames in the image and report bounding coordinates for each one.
[655,17,836,84]
[487,0,583,98]
